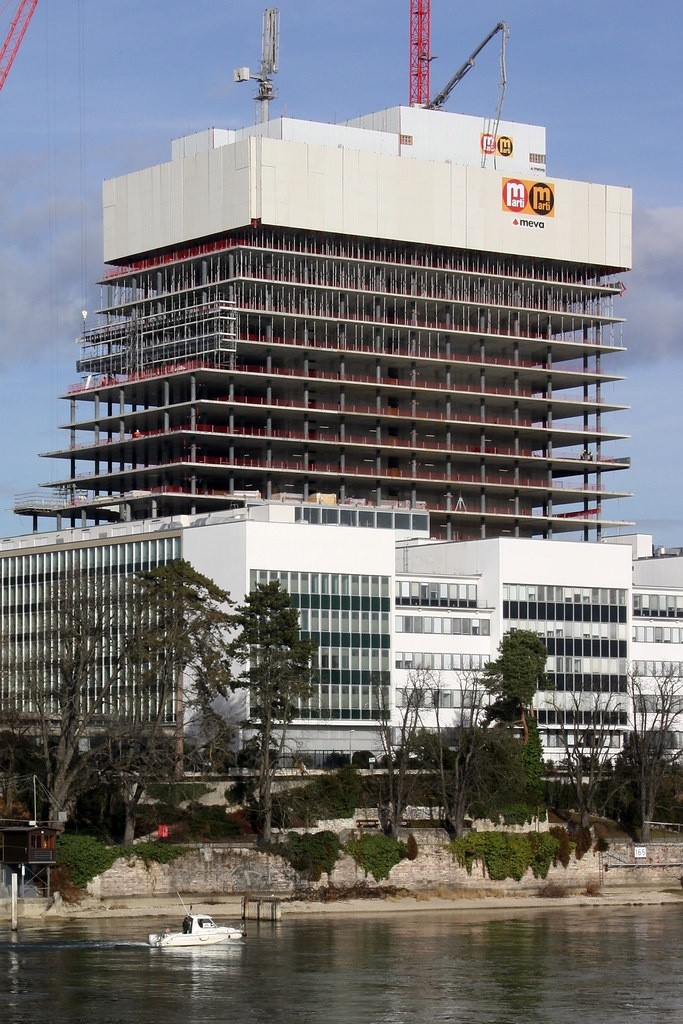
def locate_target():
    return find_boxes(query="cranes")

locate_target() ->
[408,0,432,109]
[0,0,38,92]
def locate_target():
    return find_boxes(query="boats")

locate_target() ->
[148,914,246,947]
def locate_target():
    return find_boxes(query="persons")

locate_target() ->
[182,917,190,934]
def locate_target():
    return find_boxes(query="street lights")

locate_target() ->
[349,730,355,765]
